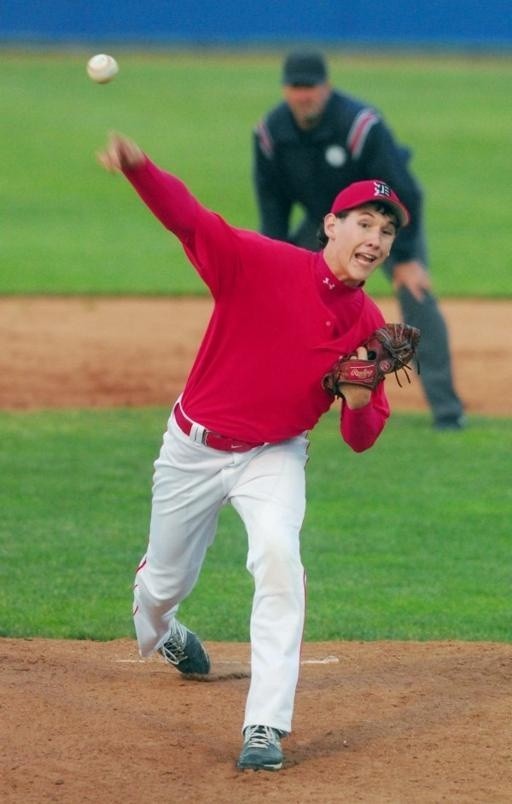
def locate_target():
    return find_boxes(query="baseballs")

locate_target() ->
[87,54,117,84]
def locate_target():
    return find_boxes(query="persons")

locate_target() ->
[92,126,410,771]
[250,49,467,430]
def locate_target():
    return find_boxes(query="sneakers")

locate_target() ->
[236,726,284,770]
[157,616,210,675]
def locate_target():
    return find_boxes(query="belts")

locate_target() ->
[174,404,263,452]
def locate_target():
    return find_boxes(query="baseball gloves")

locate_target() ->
[322,323,420,398]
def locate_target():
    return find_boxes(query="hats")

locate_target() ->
[283,49,325,86]
[330,179,410,228]
